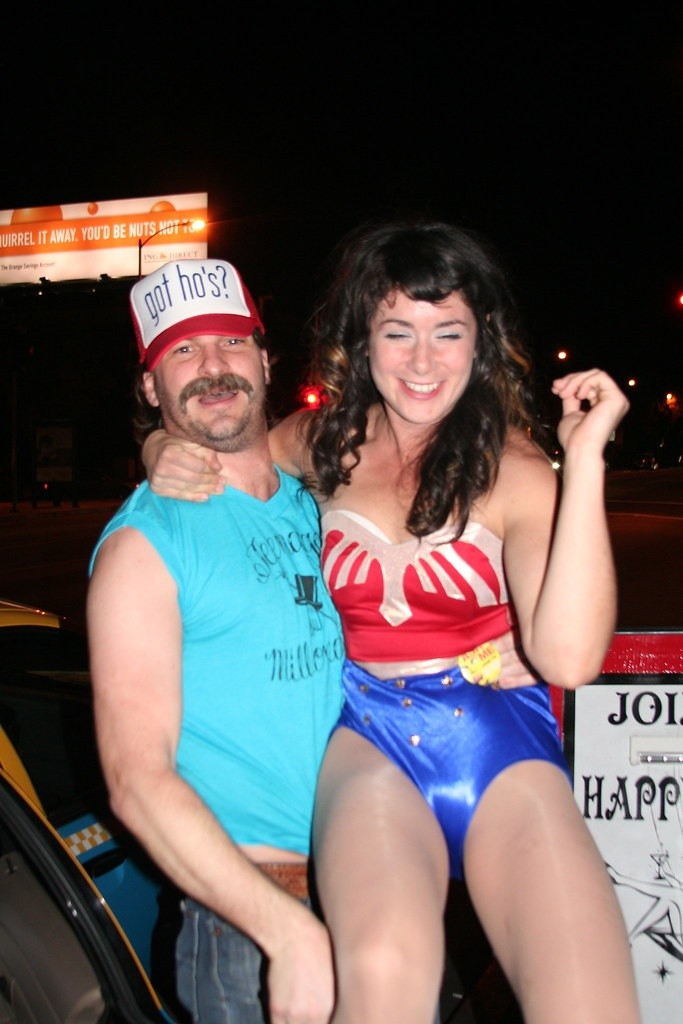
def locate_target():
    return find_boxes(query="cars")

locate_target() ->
[0,598,529,1024]
[631,453,657,470]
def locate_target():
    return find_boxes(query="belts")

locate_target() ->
[257,862,314,898]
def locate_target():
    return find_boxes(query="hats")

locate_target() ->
[128,259,265,372]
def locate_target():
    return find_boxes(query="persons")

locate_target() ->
[86,255,537,1024]
[143,219,645,1024]
